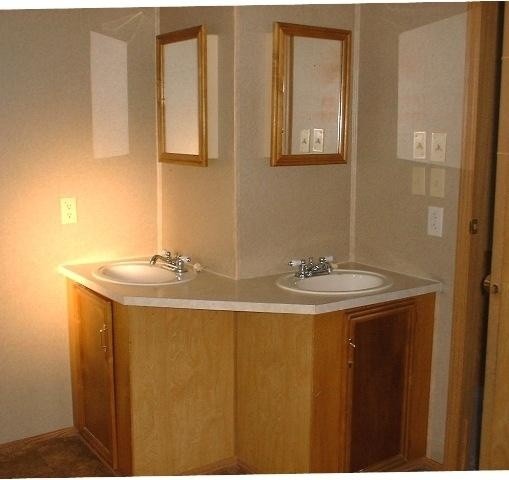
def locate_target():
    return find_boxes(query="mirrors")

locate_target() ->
[156,25,207,167]
[270,22,352,167]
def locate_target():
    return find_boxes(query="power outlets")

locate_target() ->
[411,167,426,196]
[413,132,426,161]
[60,198,78,225]
[430,168,445,199]
[427,206,443,237]
[431,133,447,163]
[313,129,324,153]
[299,129,310,153]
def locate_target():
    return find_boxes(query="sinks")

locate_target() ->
[93,258,198,286]
[282,266,394,296]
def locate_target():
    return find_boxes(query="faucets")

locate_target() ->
[149,250,192,271]
[288,254,334,279]
[174,272,186,281]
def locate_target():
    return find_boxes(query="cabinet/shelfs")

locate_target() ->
[236,292,436,471]
[66,278,235,476]
